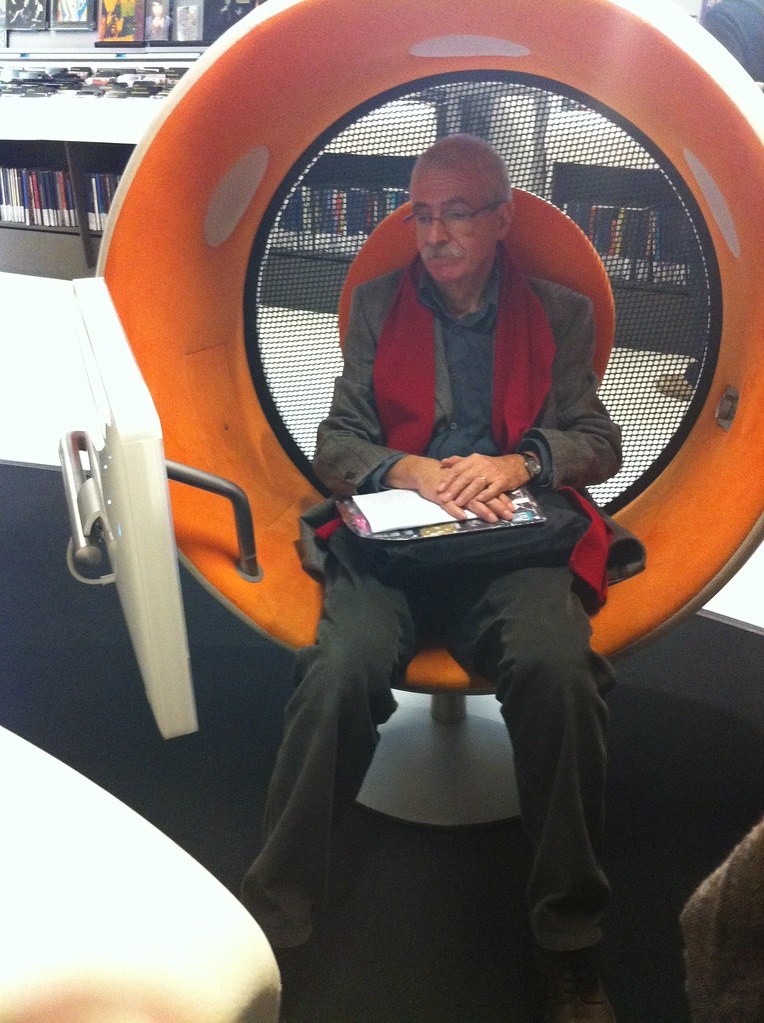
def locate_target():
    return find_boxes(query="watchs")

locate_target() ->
[521,452,541,478]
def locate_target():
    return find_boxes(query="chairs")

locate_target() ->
[96,0,763,824]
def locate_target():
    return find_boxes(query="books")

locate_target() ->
[560,200,693,287]
[82,170,124,231]
[273,183,417,255]
[0,164,79,227]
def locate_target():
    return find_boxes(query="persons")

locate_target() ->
[240,136,645,1023]
[146,0,171,40]
[654,0,764,401]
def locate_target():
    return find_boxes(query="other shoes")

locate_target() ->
[527,934,616,1023]
[271,946,296,983]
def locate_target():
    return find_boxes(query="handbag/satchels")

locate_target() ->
[334,488,547,564]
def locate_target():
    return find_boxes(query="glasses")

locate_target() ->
[402,201,499,233]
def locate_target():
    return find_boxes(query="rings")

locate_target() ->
[480,476,488,486]
